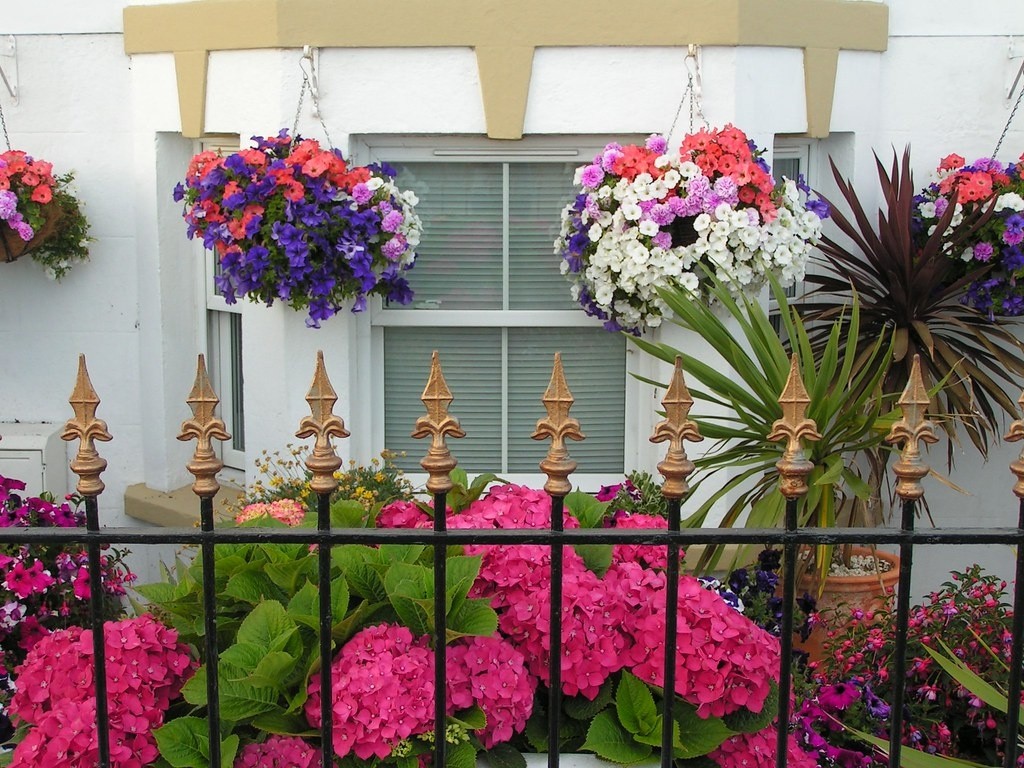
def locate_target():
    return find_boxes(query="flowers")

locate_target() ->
[553,122,831,337]
[0,149,100,285]
[172,127,424,329]
[0,440,1024,768]
[911,151,1024,322]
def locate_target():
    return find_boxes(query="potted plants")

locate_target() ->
[621,250,963,662]
